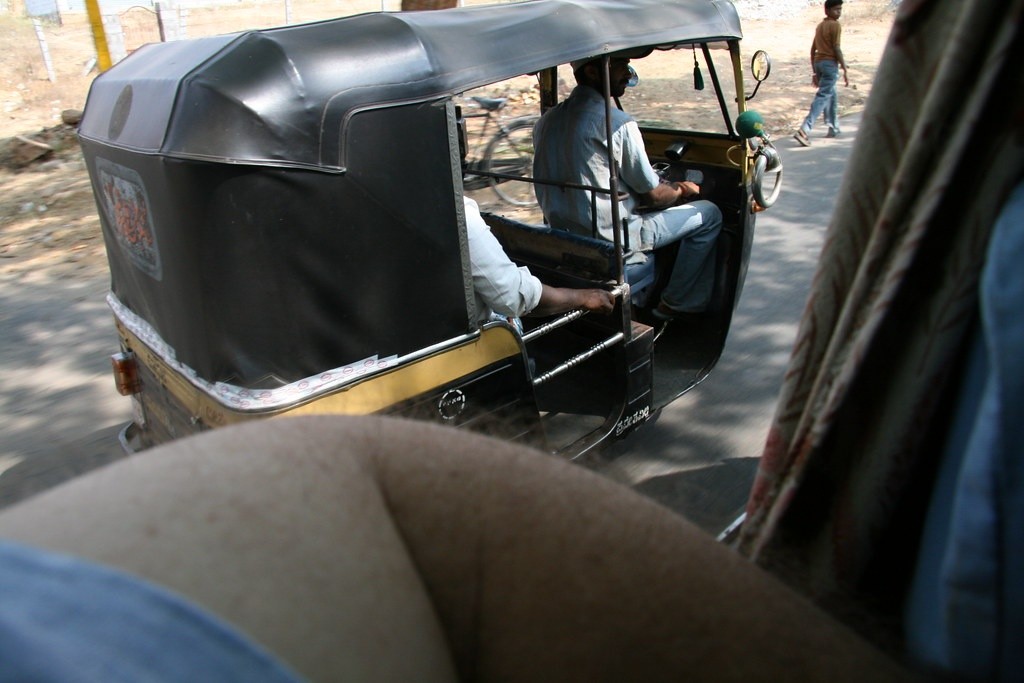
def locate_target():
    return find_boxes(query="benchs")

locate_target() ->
[479,211,655,294]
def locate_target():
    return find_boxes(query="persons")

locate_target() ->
[531,49,723,329]
[0,415,913,683]
[793,0,849,147]
[463,195,616,337]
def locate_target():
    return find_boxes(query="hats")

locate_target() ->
[570,45,654,74]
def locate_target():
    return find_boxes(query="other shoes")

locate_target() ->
[794,128,811,146]
[827,131,843,138]
[652,304,675,318]
[632,290,648,308]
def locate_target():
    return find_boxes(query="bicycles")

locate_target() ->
[462,95,548,207]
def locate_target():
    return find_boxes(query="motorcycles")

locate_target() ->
[76,0,783,467]
